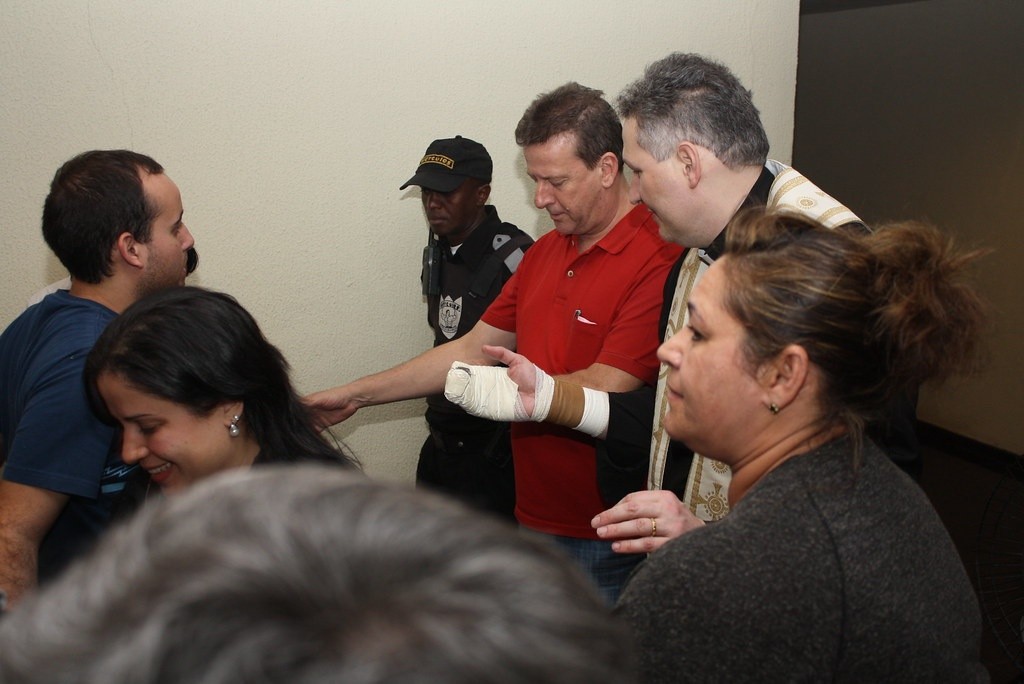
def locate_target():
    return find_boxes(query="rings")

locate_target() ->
[651,518,656,536]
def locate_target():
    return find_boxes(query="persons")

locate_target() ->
[83,287,360,494]
[300,81,684,604]
[443,51,918,558]
[606,207,988,684]
[0,458,635,684]
[399,135,537,531]
[0,148,200,615]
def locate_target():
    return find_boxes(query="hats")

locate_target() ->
[400,134,493,193]
